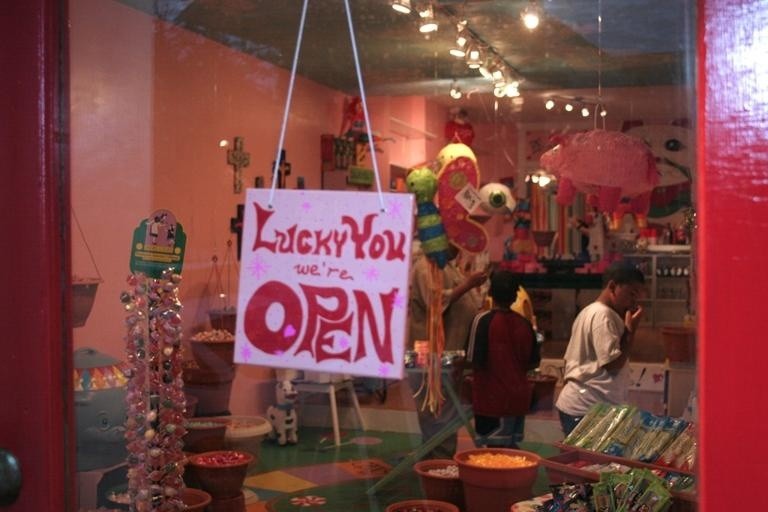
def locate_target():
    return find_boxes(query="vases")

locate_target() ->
[384,448,544,512]
[657,323,696,369]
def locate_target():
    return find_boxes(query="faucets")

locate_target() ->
[665,231,673,243]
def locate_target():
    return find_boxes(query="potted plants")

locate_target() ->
[68,206,105,329]
[153,239,274,512]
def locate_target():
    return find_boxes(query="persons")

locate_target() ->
[408,239,543,462]
[556,256,646,437]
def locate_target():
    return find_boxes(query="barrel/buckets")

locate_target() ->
[659,323,692,361]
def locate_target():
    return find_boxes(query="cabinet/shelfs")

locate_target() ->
[619,255,694,328]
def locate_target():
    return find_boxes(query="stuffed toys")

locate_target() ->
[262,379,299,446]
[339,96,662,274]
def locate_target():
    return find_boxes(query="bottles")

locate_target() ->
[664,222,685,245]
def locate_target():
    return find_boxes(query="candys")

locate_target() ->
[390,453,535,512]
[187,395,259,464]
[108,268,186,512]
[193,328,234,340]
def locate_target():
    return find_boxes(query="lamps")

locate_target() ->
[389,0,439,34]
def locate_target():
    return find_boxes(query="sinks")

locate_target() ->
[646,243,691,252]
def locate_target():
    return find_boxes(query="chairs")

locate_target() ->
[275,368,368,449]
